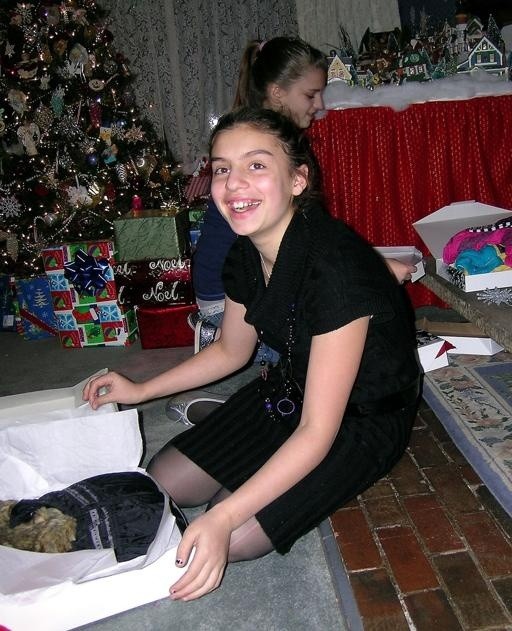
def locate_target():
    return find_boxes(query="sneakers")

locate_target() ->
[165,391,230,427]
[187,312,202,330]
[195,321,217,355]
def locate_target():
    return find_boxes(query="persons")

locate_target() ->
[81,103,423,603]
[187,32,416,365]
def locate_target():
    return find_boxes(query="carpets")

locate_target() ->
[416,333,512,519]
[0,332,365,631]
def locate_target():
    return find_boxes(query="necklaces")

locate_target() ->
[259,253,273,279]
[256,301,296,425]
